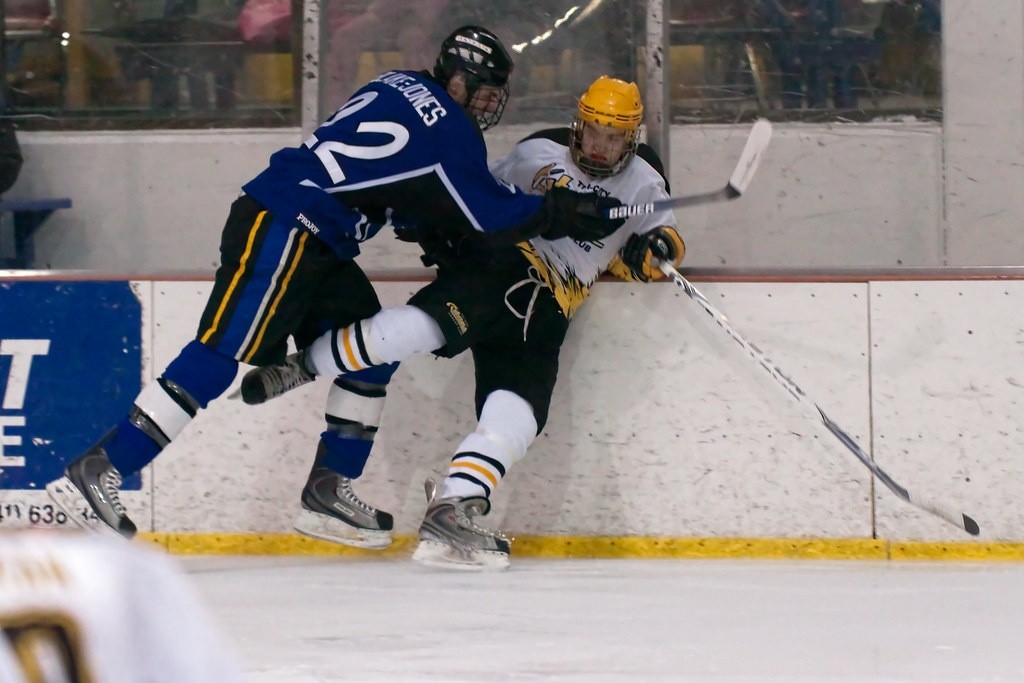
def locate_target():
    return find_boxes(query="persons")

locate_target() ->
[41,28,630,543]
[313,0,459,113]
[0,524,246,683]
[229,74,683,571]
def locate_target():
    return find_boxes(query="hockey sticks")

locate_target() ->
[609,118,775,220]
[654,256,980,538]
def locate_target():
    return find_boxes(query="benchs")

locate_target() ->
[0,198,72,269]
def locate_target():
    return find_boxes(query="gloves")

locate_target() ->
[538,187,628,242]
[631,223,686,282]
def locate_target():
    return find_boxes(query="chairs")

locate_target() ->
[0,0,865,128]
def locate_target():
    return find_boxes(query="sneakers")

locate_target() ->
[46,449,137,540]
[411,479,510,571]
[293,465,393,550]
[228,347,317,406]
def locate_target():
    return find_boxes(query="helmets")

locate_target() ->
[567,75,645,144]
[432,25,514,98]
